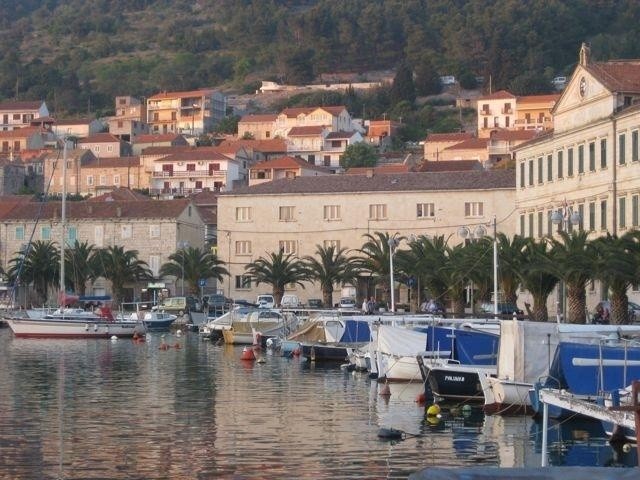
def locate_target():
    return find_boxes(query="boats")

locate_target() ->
[41,306,103,323]
[202,306,640,422]
[119,309,178,330]
[0,279,19,328]
[27,306,61,321]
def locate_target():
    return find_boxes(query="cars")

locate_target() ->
[479,292,525,321]
[416,300,446,320]
[191,294,232,314]
[304,299,325,312]
[590,301,640,325]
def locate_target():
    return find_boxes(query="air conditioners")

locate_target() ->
[197,161,204,165]
[177,161,183,166]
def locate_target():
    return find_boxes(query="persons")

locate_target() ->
[362,296,377,315]
[84,301,115,322]
[592,306,610,324]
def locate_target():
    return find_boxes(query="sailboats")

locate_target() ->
[5,136,148,341]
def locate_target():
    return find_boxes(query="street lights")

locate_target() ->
[551,197,580,323]
[387,235,401,316]
[457,224,486,321]
[407,233,432,315]
[191,102,199,133]
[175,240,191,296]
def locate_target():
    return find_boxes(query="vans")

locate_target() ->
[440,76,455,85]
[256,294,277,311]
[151,296,195,318]
[280,294,299,313]
[551,76,567,85]
[335,296,358,313]
